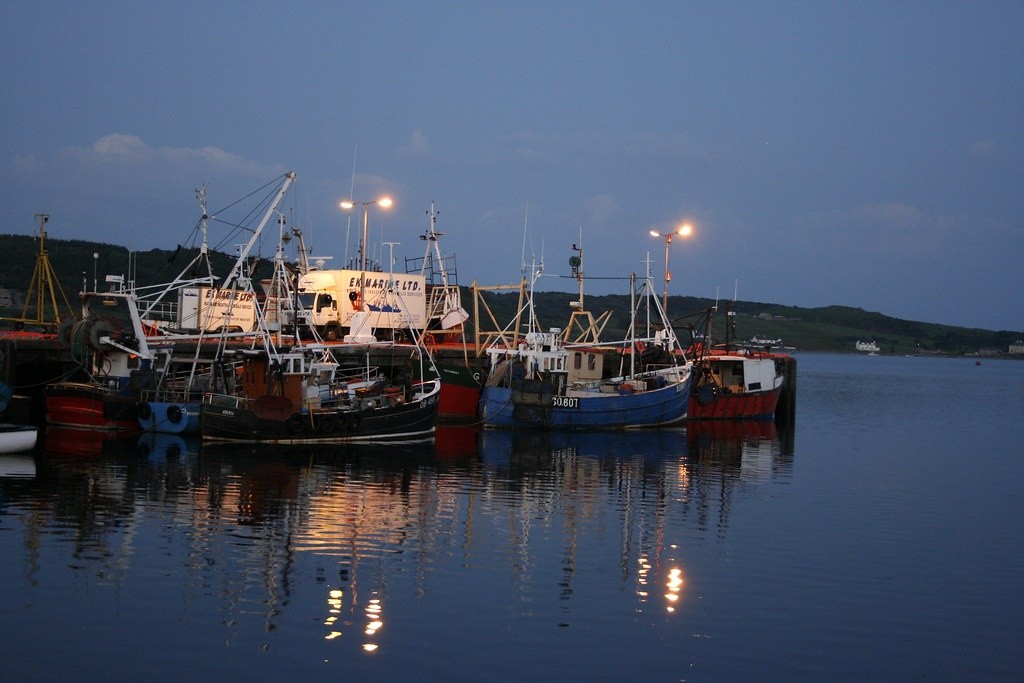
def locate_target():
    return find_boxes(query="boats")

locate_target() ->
[42,149,495,454]
[473,206,699,434]
[681,274,798,420]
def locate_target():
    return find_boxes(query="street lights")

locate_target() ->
[650,228,691,317]
[343,198,392,311]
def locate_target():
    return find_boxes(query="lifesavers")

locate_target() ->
[306,413,320,433]
[349,412,363,431]
[288,412,306,432]
[166,405,182,423]
[334,412,349,430]
[320,414,335,433]
[137,401,151,420]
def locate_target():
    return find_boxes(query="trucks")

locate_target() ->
[294,269,426,343]
